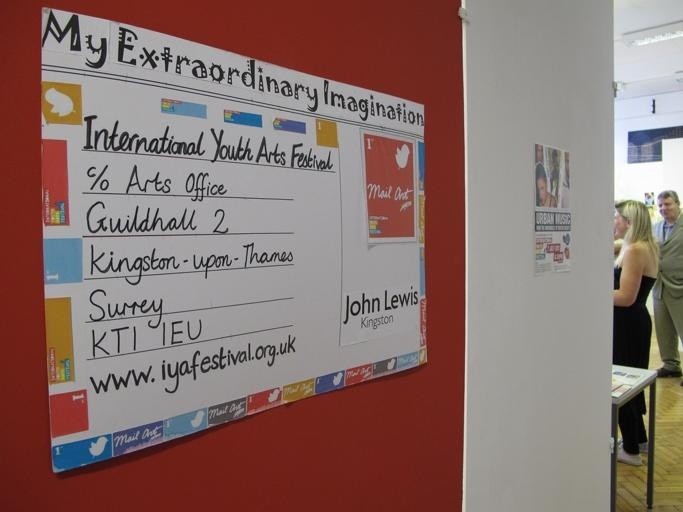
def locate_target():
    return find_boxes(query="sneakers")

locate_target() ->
[617,438,648,465]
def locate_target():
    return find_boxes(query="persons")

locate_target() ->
[651,190,683,387]
[613,199,658,466]
[536,163,557,208]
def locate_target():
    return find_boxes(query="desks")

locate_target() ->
[608,362,659,511]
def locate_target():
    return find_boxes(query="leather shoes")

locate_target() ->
[655,366,682,379]
